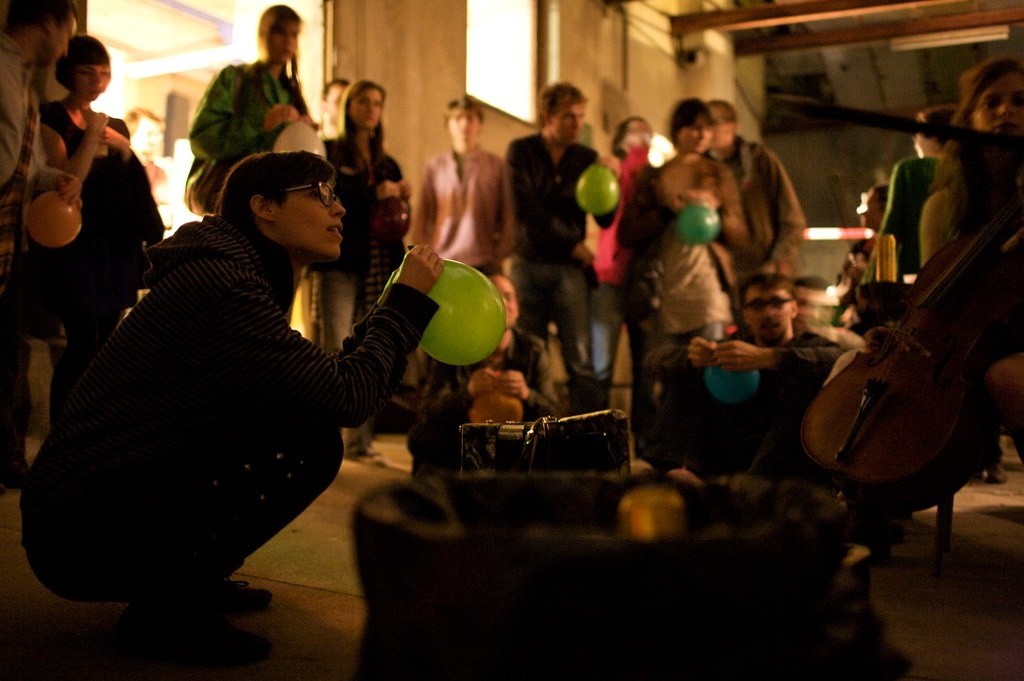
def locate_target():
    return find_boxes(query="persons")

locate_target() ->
[429,261,561,421]
[647,271,844,479]
[619,98,746,347]
[704,101,807,327]
[593,116,653,284]
[0,0,76,493]
[188,5,320,327]
[323,80,413,465]
[506,82,625,416]
[20,136,441,665]
[831,54,1024,484]
[413,94,515,414]
[41,34,166,436]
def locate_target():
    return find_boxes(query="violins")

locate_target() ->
[800,186,1024,506]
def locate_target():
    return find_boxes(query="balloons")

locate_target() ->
[704,366,759,403]
[576,163,619,216]
[470,370,523,424]
[371,196,411,238]
[419,258,506,365]
[27,181,82,247]
[678,203,721,243]
[272,116,325,158]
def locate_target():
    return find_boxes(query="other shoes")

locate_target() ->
[983,464,1008,481]
[112,608,273,668]
[215,577,272,612]
[347,441,380,457]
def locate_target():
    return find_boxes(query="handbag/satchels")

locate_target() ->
[370,197,411,242]
[184,66,229,217]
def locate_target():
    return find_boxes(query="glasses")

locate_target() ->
[280,179,343,208]
[743,294,794,311]
